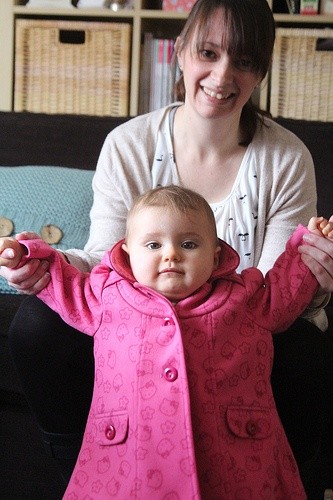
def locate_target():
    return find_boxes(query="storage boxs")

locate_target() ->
[268,26,333,125]
[11,16,130,118]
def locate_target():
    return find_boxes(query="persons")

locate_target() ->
[0,183,332,499]
[0,0,332,485]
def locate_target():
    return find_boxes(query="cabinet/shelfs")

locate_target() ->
[0,0,333,125]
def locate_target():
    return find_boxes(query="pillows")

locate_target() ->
[1,163,96,291]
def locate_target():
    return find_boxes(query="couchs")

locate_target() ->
[0,108,333,500]
